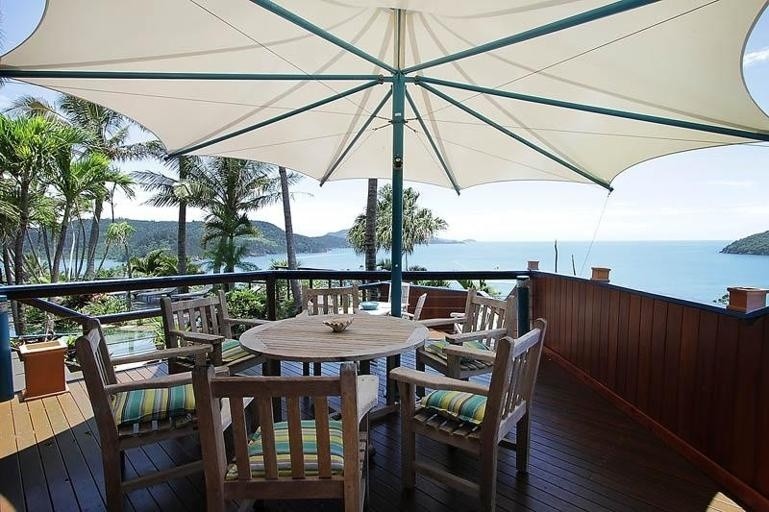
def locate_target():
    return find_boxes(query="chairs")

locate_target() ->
[188,365,381,512]
[78,318,256,512]
[390,318,548,512]
[411,287,521,401]
[300,285,372,404]
[397,292,427,323]
[159,290,279,440]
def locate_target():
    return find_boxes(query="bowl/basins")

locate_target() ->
[360,302,379,310]
[323,318,355,333]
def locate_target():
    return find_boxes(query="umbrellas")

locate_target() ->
[0,0,769,405]
